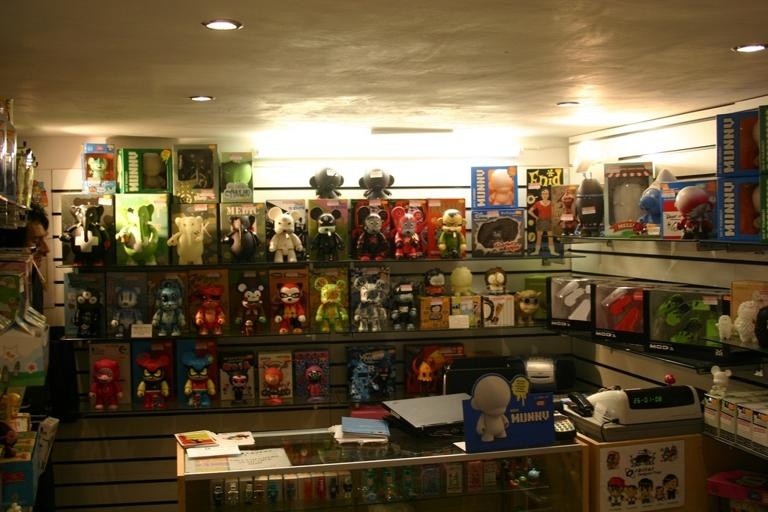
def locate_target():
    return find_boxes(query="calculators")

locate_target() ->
[550,414,576,440]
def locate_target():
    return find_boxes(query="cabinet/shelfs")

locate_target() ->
[175,417,590,512]
[542,234,768,374]
[56,255,587,419]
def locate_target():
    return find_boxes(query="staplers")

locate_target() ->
[568,391,593,417]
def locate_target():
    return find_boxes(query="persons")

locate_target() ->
[4,202,51,316]
